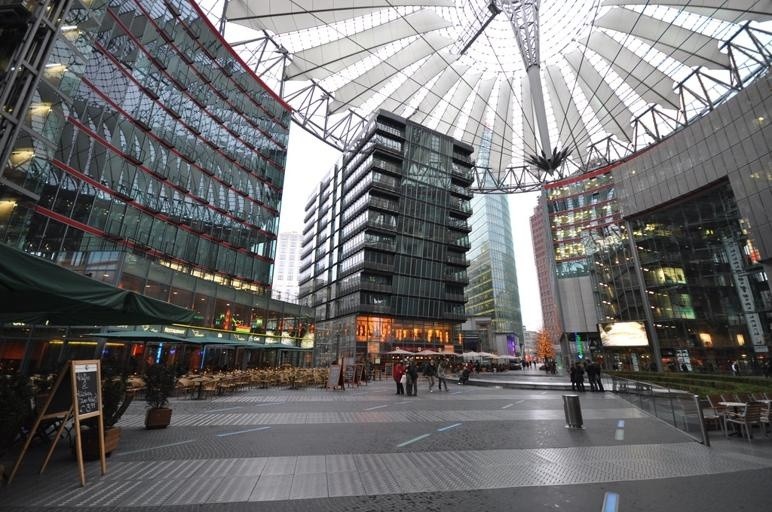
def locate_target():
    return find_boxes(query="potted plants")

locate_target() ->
[73,362,134,459]
[140,364,177,429]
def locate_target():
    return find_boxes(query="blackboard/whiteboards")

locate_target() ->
[357,365,363,382]
[385,363,393,378]
[345,365,357,384]
[70,359,103,420]
[327,365,341,386]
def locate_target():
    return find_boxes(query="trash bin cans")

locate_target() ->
[563,395,584,430]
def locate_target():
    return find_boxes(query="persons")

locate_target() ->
[570,357,605,392]
[458,359,481,385]
[545,359,556,374]
[522,360,531,367]
[392,355,449,396]
[727,359,741,376]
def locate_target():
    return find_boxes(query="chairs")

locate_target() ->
[706,390,772,444]
[111,364,344,398]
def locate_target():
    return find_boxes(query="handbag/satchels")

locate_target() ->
[400,375,406,383]
